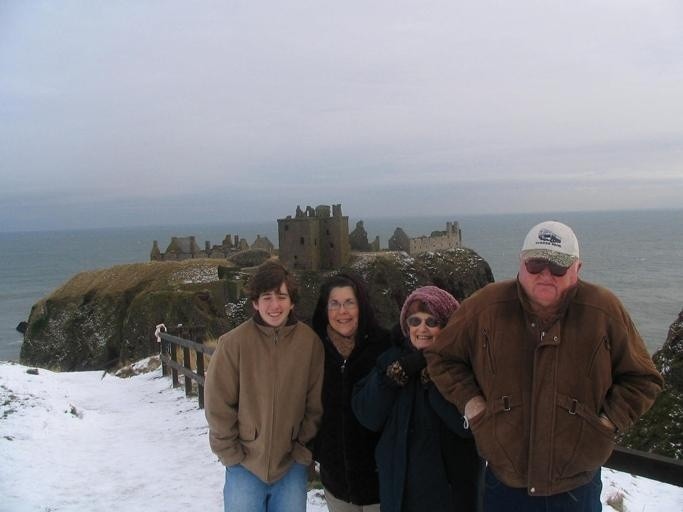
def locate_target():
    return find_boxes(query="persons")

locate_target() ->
[420,220,665,512]
[347,285,487,511]
[303,270,395,512]
[201,263,329,512]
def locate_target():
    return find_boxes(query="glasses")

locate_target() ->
[406,317,441,328]
[525,259,569,279]
[326,300,360,310]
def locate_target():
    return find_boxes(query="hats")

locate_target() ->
[399,286,460,338]
[522,220,580,268]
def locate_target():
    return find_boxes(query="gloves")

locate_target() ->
[398,348,428,374]
